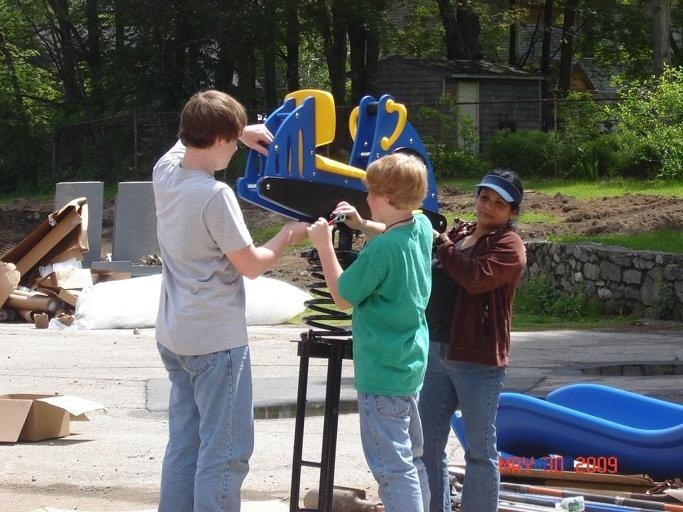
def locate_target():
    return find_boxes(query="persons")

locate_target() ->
[417,166,530,510]
[305,149,437,512]
[148,89,315,512]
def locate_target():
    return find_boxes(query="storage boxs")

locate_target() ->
[0,394,105,444]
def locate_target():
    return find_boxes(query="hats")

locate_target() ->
[473,173,521,203]
